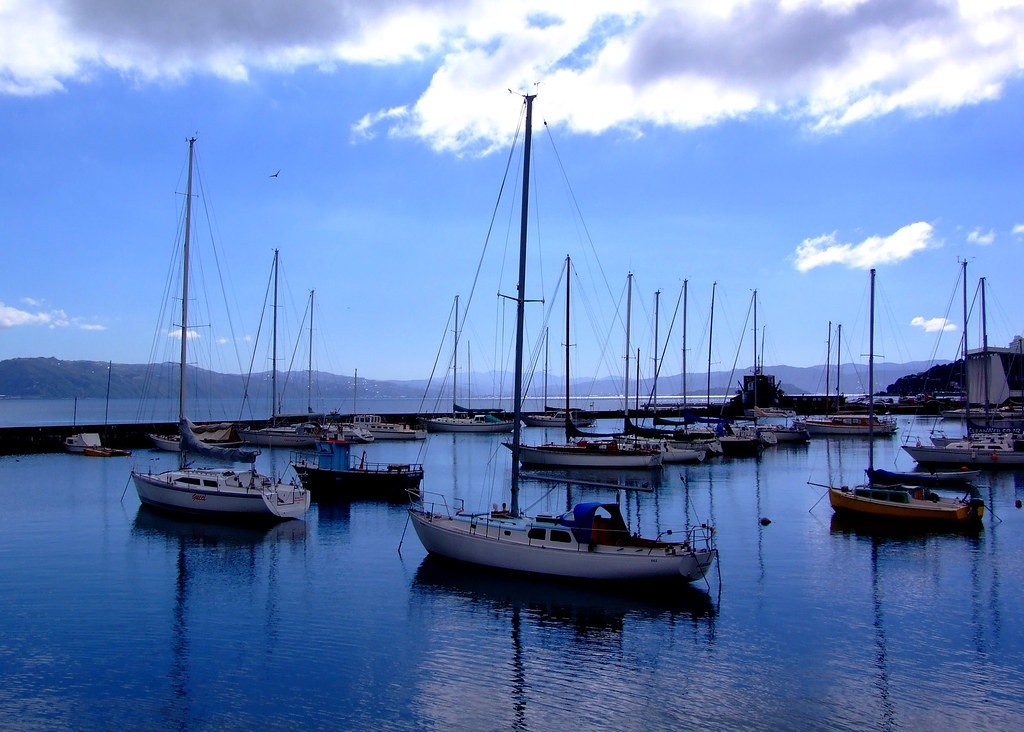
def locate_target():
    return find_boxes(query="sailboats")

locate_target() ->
[132,135,312,519]
[827,268,985,526]
[151,247,1023,502]
[404,81,718,585]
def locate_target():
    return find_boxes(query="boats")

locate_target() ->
[83,447,131,458]
[64,432,101,454]
